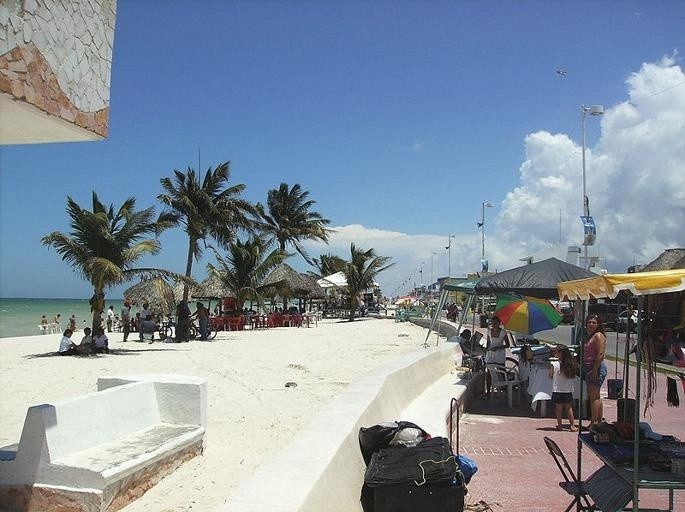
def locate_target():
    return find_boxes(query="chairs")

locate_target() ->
[543,435,598,512]
[109,309,324,332]
[487,363,522,409]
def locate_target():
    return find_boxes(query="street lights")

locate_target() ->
[477,200,496,315]
[394,251,437,300]
[580,104,604,269]
[445,234,456,283]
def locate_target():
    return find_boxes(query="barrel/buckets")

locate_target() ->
[608,371,623,400]
[616,388,635,423]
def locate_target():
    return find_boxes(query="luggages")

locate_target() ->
[361,437,465,512]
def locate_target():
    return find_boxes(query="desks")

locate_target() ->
[578,429,685,512]
[525,354,590,420]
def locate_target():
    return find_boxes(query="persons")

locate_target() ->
[484,315,510,399]
[518,344,532,378]
[577,313,608,430]
[549,345,579,431]
[459,328,486,357]
[37,297,211,356]
[408,299,417,312]
[400,299,409,311]
[211,305,308,328]
[446,302,461,323]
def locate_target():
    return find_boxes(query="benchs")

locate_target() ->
[41,376,208,511]
[0,375,142,511]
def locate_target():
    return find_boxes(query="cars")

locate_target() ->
[487,305,497,317]
[478,303,489,314]
[616,310,656,334]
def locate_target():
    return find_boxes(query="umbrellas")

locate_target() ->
[394,296,425,315]
[190,270,241,318]
[473,258,603,300]
[253,262,349,311]
[121,274,178,327]
[492,291,564,337]
[167,274,209,312]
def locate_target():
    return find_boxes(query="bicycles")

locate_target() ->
[159,315,218,341]
